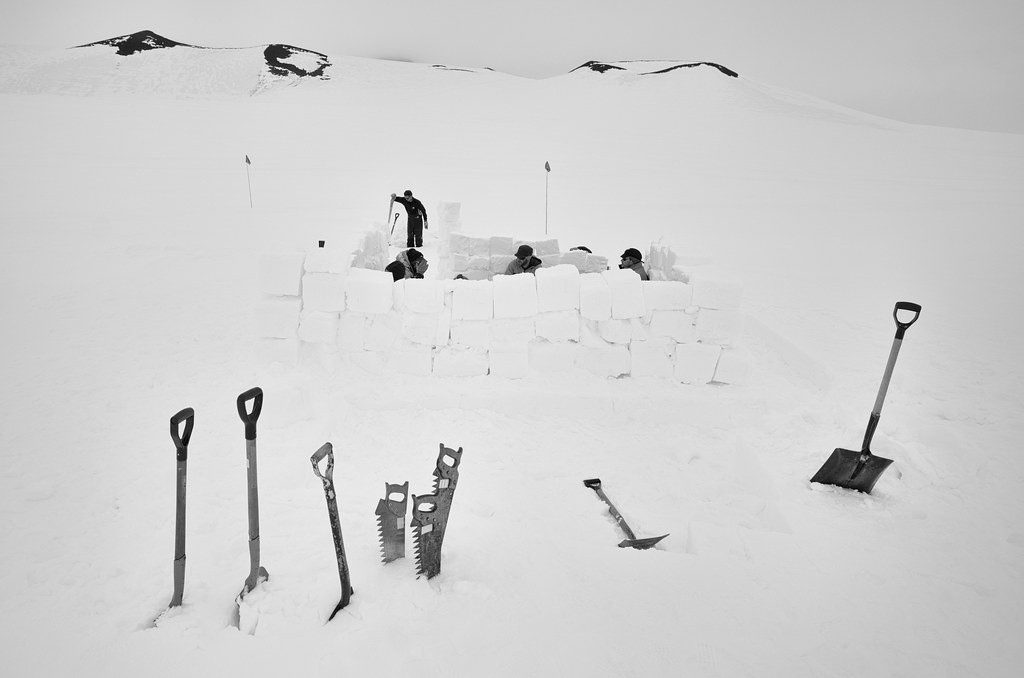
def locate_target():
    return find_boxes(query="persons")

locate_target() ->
[618,248,649,280]
[391,190,429,248]
[384,248,429,283]
[504,245,542,275]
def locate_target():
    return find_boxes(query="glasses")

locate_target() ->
[405,198,410,200]
[622,258,633,261]
[518,257,527,260]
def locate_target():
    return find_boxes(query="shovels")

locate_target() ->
[811,301,921,496]
[582,476,671,550]
[152,406,197,628]
[235,387,269,630]
[311,442,354,623]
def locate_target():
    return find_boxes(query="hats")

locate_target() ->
[620,248,642,261]
[385,261,405,282]
[407,248,421,260]
[514,245,533,259]
